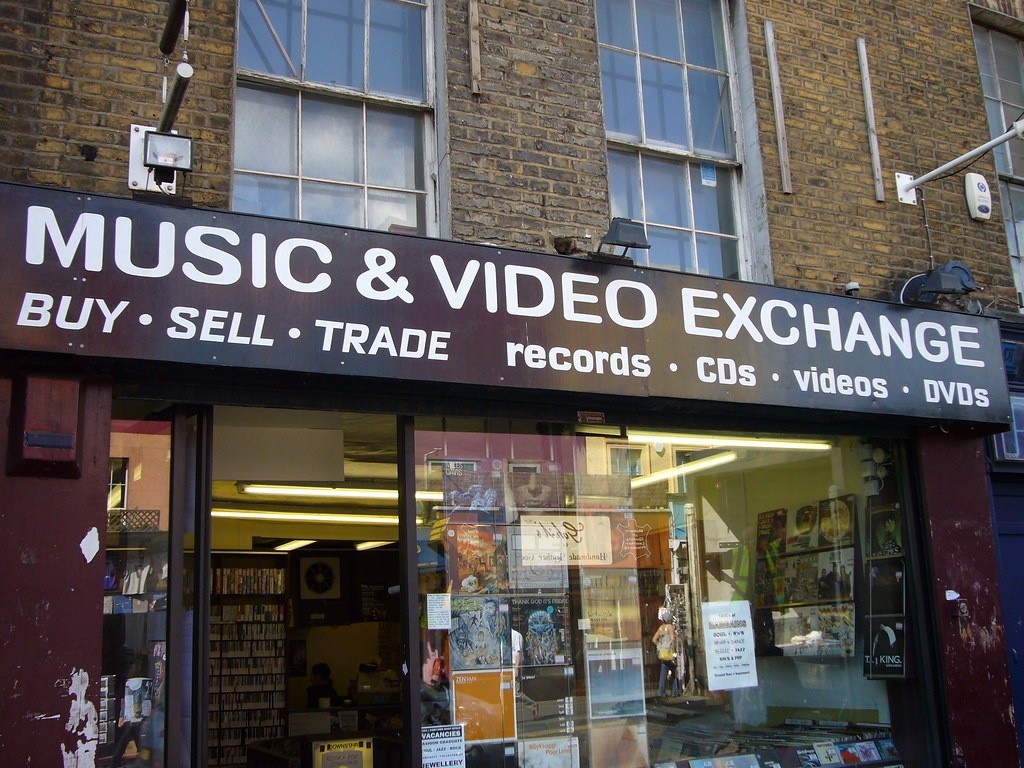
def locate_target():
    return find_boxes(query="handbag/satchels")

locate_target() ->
[659,624,677,660]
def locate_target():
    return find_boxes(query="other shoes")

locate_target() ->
[657,694,668,698]
[673,694,681,697]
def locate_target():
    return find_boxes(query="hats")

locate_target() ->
[662,613,673,623]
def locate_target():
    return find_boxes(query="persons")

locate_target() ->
[816,549,855,602]
[498,613,539,721]
[287,663,338,713]
[457,599,506,665]
[652,608,682,699]
[108,651,149,768]
[506,472,558,523]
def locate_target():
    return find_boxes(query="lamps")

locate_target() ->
[914,273,965,310]
[142,130,193,182]
[586,218,652,266]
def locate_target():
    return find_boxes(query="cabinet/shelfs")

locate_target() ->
[208,554,291,768]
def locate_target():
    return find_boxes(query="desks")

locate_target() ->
[247,723,413,768]
